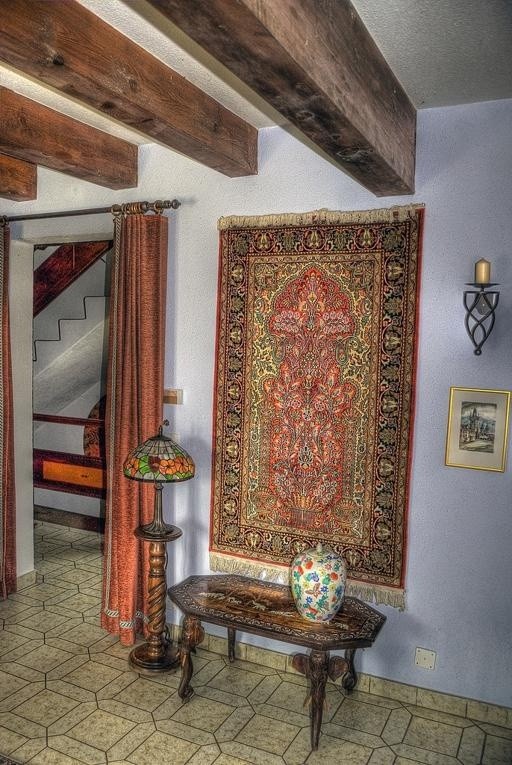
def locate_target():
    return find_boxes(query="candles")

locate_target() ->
[475,257,492,283]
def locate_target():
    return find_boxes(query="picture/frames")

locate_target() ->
[443,387,512,474]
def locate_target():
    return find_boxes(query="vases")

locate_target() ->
[290,539,348,624]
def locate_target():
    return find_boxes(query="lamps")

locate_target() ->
[120,421,196,677]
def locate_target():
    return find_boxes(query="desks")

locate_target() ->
[161,572,386,751]
